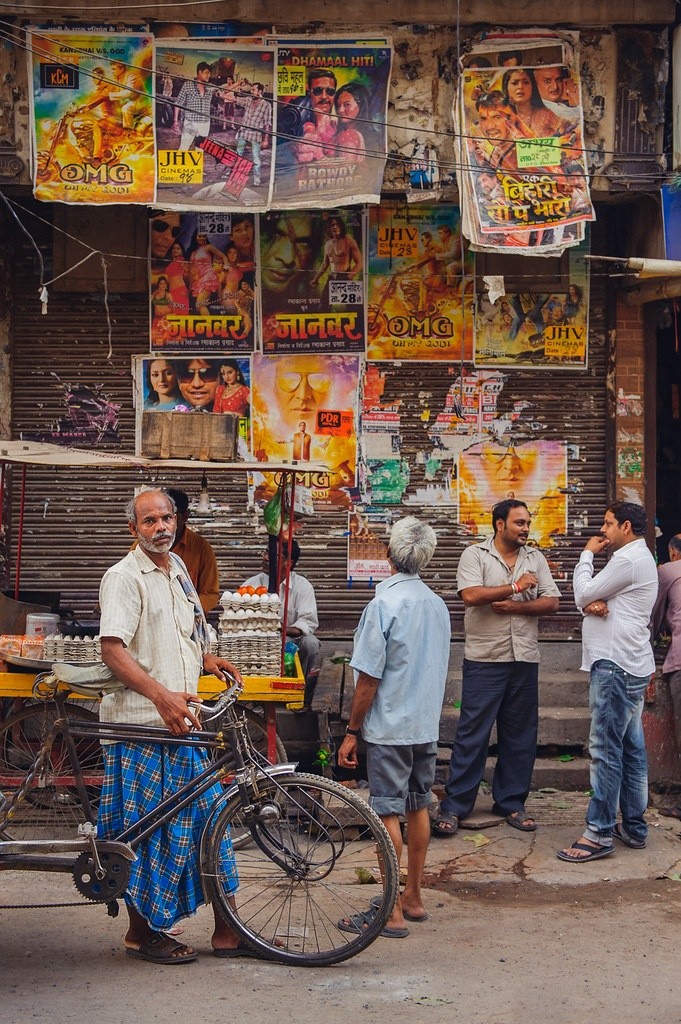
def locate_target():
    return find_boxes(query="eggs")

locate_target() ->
[237,585,267,595]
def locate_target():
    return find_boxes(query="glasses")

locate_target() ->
[151,218,183,238]
[176,366,219,383]
[260,550,286,560]
[312,85,337,96]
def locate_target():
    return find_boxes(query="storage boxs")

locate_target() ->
[140,410,237,463]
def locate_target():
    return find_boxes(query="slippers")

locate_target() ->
[214,931,292,960]
[126,932,199,964]
[339,905,411,938]
[556,841,617,862]
[610,822,648,848]
[371,895,428,923]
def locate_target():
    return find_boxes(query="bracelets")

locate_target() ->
[509,582,520,593]
[345,725,361,735]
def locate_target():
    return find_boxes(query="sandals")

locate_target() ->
[433,809,458,834]
[506,808,537,831]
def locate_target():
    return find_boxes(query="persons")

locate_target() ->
[26,25,589,490]
[240,537,322,714]
[336,515,452,938]
[557,502,659,863]
[650,534,681,817]
[426,499,562,839]
[126,488,220,619]
[94,491,287,965]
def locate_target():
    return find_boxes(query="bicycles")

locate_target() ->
[0,694,291,851]
[0,663,399,969]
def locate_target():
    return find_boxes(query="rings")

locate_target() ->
[595,606,598,609]
